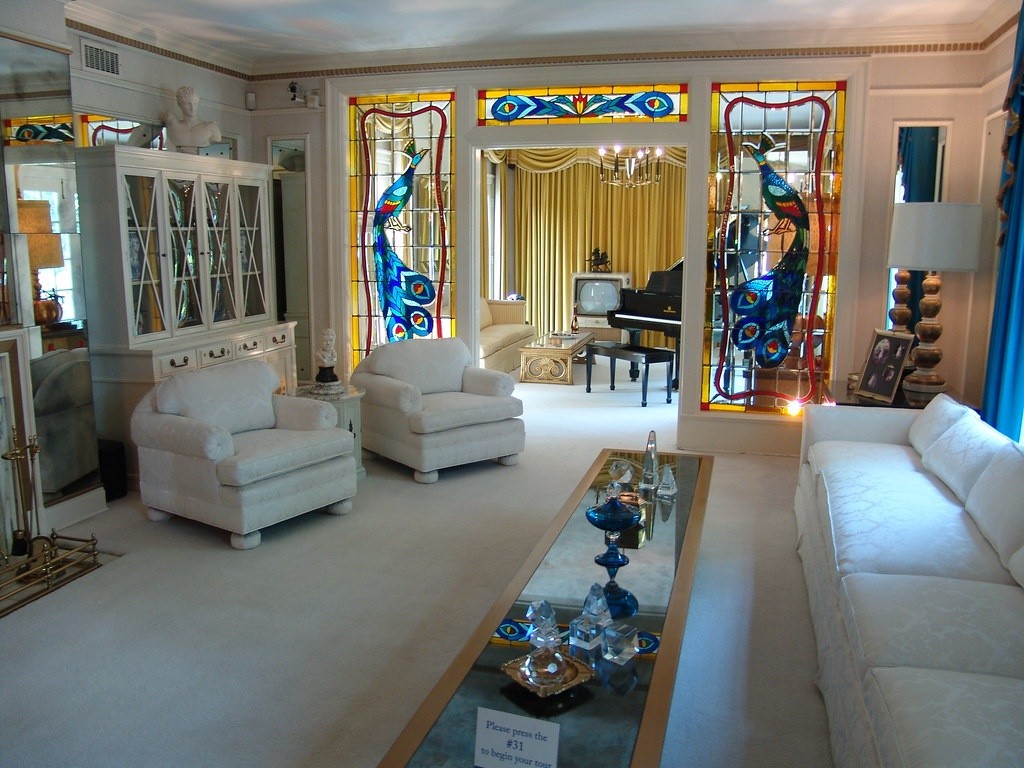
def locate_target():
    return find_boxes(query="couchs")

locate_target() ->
[427,297,536,374]
[130,358,360,550]
[349,336,526,484]
[30,348,99,501]
[793,404,1024,768]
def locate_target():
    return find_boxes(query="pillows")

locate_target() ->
[920,409,1013,505]
[964,440,1024,569]
[907,392,981,456]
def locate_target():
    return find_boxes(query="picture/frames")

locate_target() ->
[853,327,915,404]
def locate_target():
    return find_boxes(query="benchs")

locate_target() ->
[584,341,675,407]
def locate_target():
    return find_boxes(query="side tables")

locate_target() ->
[294,385,367,481]
[821,379,982,416]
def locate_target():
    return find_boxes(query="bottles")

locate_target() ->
[571,303,579,334]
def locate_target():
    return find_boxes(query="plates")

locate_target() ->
[551,335,575,339]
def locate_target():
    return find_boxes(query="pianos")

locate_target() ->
[605,214,767,392]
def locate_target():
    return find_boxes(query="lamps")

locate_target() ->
[27,233,64,302]
[886,202,984,393]
[17,200,53,234]
[598,144,664,191]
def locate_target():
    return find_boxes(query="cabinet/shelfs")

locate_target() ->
[278,172,311,380]
[73,145,297,491]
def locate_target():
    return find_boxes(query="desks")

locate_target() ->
[577,317,630,344]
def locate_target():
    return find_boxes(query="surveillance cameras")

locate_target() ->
[289,80,301,94]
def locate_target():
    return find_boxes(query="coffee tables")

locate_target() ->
[375,448,715,768]
[517,331,597,386]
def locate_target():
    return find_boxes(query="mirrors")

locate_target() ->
[266,132,315,385]
[885,120,955,370]
[0,37,104,509]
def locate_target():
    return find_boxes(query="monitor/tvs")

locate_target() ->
[572,272,632,328]
